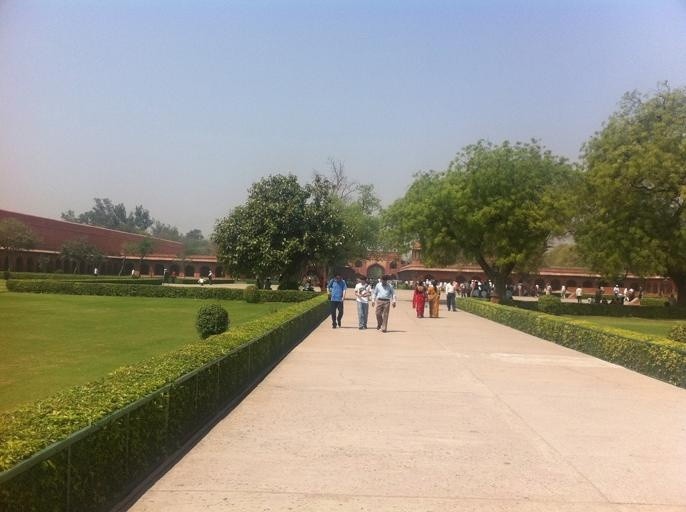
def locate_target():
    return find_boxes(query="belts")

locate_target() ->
[377,299,389,300]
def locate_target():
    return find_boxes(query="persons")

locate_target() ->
[131,269,135,275]
[445,279,457,311]
[254,278,265,290]
[163,268,169,283]
[93,267,98,276]
[412,280,428,318]
[327,273,347,328]
[365,277,645,305]
[170,271,177,284]
[264,278,272,290]
[668,294,676,305]
[371,276,397,333]
[354,275,372,330]
[302,279,315,292]
[197,277,206,285]
[208,270,213,285]
[427,280,441,318]
